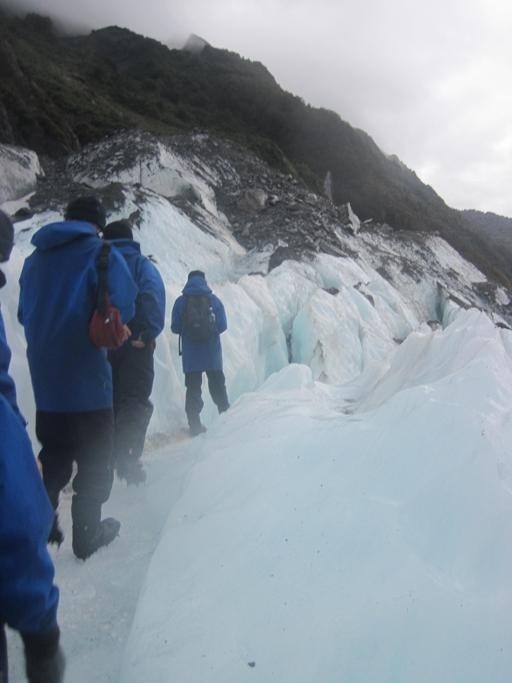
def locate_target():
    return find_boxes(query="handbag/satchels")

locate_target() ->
[89,306,128,348]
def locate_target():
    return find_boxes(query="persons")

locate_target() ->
[15,194,139,557]
[0,209,69,682]
[99,218,165,487]
[171,269,232,435]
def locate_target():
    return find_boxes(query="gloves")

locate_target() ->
[21,628,64,683]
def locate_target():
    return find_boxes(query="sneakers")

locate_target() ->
[188,426,207,436]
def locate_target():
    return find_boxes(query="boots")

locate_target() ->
[118,461,147,485]
[72,511,121,559]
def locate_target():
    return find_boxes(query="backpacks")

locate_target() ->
[182,295,216,341]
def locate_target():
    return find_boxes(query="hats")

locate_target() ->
[0,210,14,262]
[64,197,106,231]
[102,222,133,240]
